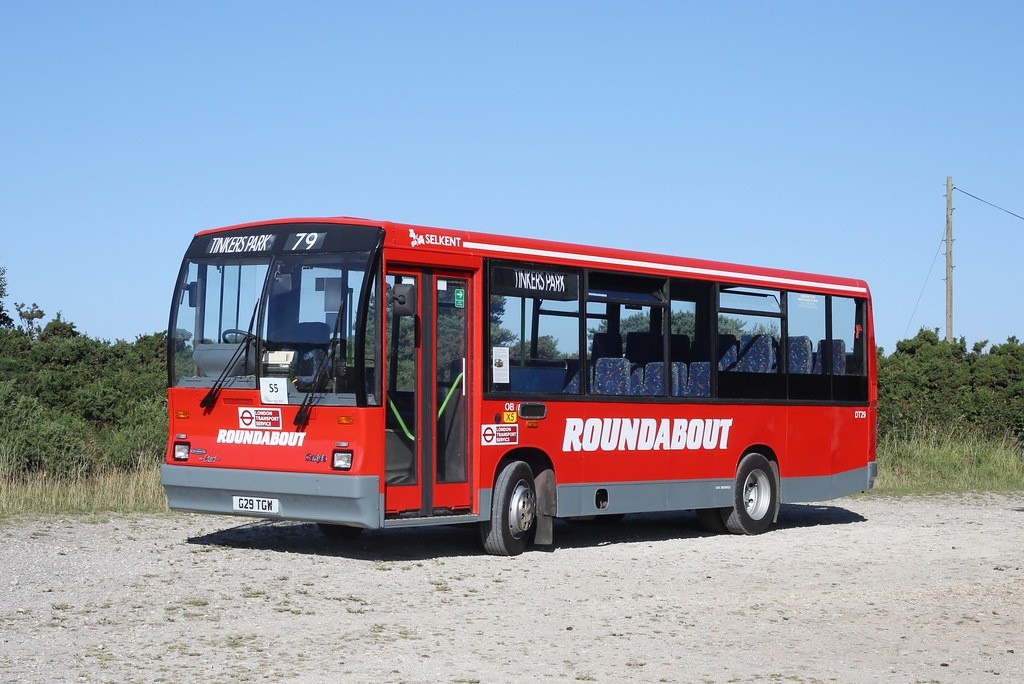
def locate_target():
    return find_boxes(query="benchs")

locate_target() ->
[490,355,567,392]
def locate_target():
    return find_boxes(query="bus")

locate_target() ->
[162,216,878,556]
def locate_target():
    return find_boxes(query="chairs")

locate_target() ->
[563,358,594,394]
[739,335,772,373]
[779,336,814,374]
[641,362,688,396]
[631,363,644,395]
[626,332,659,362]
[813,339,846,375]
[593,357,630,395]
[659,333,690,362]
[687,362,723,396]
[719,334,738,371]
[290,322,330,377]
[591,332,622,365]
